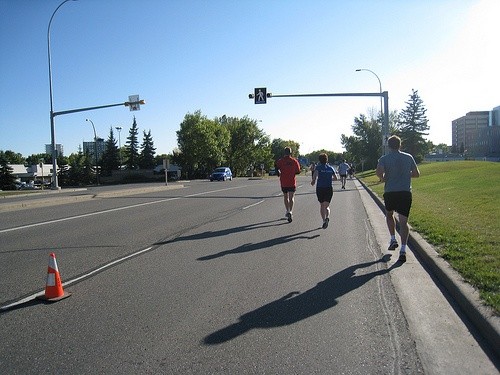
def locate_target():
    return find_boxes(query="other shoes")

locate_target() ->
[287,212,292,223]
[323,218,329,229]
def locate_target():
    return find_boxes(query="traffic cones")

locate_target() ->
[35,253,73,303]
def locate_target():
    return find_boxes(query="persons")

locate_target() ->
[275,147,301,222]
[304,159,355,190]
[376,136,420,262]
[311,153,338,229]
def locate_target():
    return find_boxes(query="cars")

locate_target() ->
[210,167,233,182]
[269,168,277,176]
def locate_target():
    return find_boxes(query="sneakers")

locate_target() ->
[388,239,399,250]
[399,251,406,262]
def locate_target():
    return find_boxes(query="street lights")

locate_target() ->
[85,118,100,185]
[356,68,383,129]
